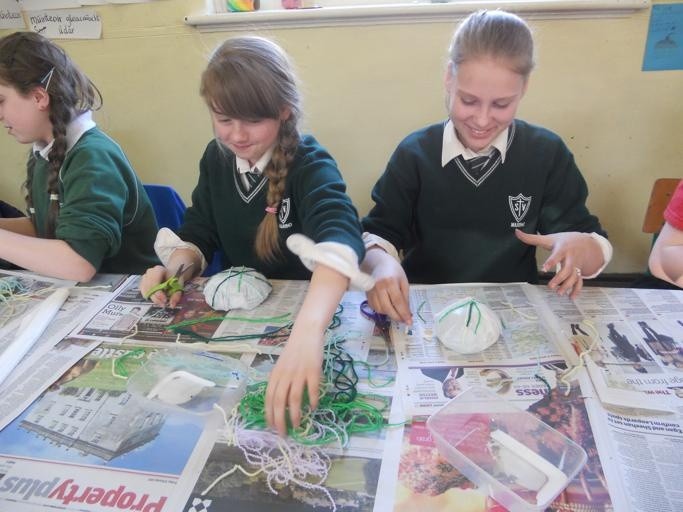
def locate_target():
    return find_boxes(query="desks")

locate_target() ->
[2,279,681,511]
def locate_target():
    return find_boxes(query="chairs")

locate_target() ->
[642,179,681,289]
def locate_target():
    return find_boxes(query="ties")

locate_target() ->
[246,172,262,190]
[468,156,489,177]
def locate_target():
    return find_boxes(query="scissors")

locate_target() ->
[144,261,196,303]
[360,300,392,348]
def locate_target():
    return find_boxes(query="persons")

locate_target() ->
[421,368,464,399]
[573,321,683,373]
[0,31,164,282]
[139,35,366,440]
[658,180,683,282]
[359,11,613,326]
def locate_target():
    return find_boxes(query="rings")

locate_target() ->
[576,267,581,276]
